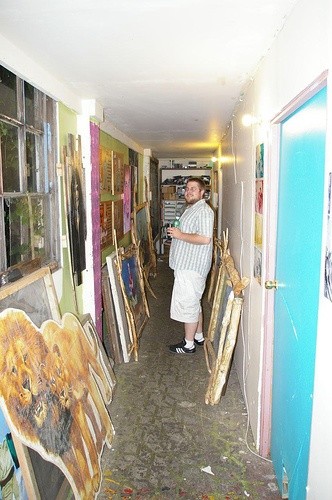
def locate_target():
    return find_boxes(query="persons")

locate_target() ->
[168,178,215,356]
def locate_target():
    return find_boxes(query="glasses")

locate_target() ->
[185,187,201,191]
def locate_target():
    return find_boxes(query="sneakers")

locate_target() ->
[194,338,205,347]
[169,338,196,354]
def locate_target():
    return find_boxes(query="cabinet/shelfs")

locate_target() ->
[158,167,213,255]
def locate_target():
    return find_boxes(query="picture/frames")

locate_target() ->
[105,246,135,363]
[100,266,124,365]
[114,248,150,345]
[133,201,156,276]
[66,165,86,273]
[0,265,75,500]
[77,313,118,408]
[203,227,248,406]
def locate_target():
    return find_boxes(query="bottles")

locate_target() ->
[173,217,180,238]
[166,220,173,240]
